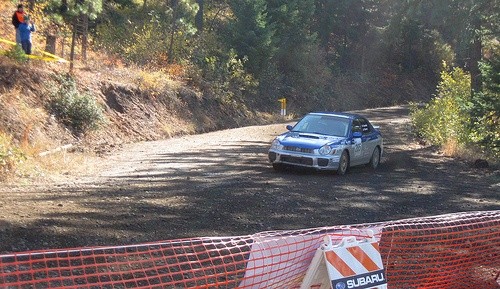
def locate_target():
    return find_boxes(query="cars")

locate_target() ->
[268,111,384,175]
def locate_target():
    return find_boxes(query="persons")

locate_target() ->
[19,15,35,54]
[12,3,27,46]
